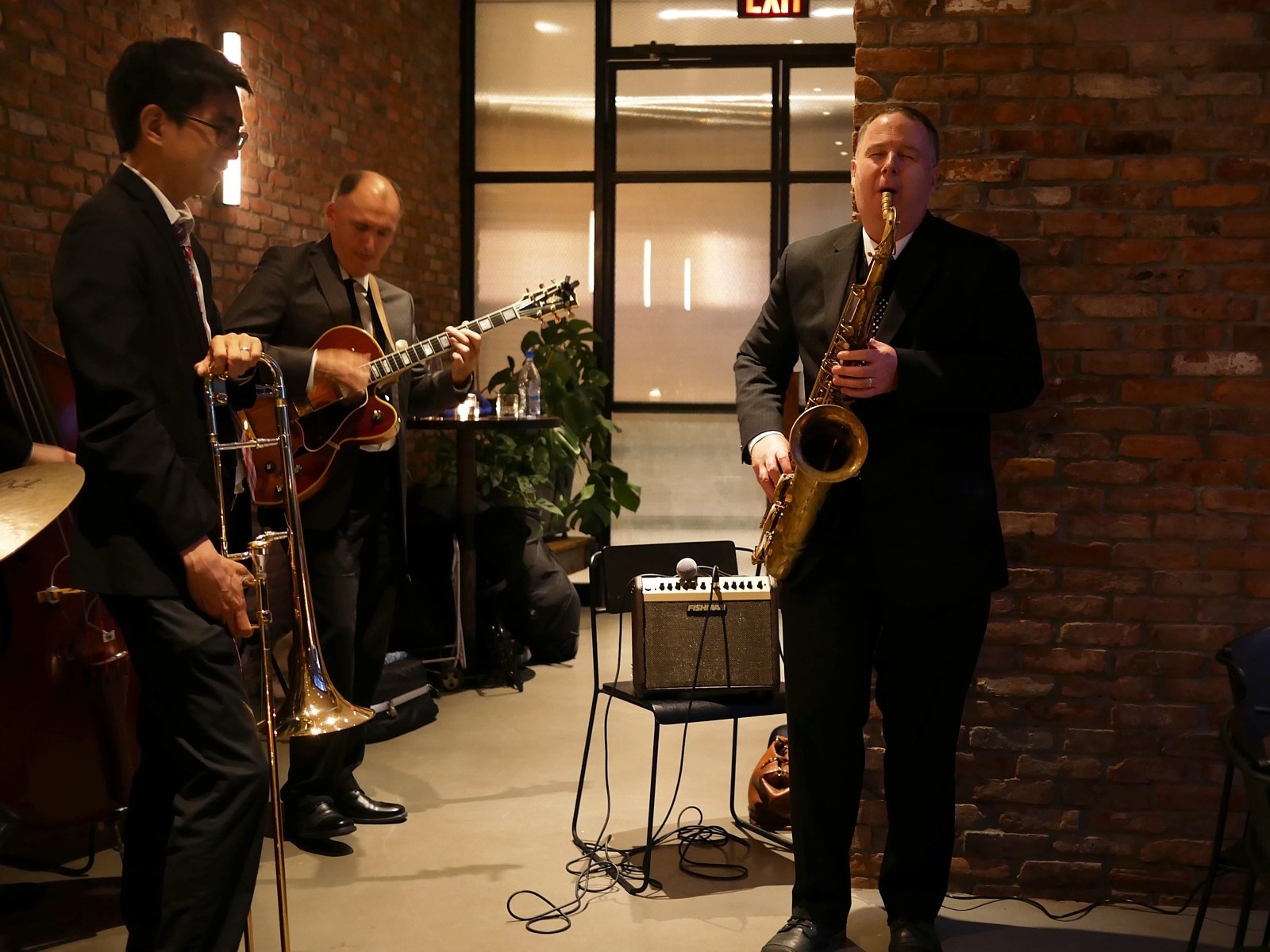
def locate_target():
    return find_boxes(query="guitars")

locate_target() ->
[238,274,582,507]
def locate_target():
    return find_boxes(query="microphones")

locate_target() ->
[675,556,697,579]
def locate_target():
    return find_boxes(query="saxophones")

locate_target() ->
[751,191,900,580]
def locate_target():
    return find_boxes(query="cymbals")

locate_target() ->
[0,461,88,561]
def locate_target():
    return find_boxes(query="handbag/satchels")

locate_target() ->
[354,674,438,742]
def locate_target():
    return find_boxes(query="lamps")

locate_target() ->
[223,32,244,207]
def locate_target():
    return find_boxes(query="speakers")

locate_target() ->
[632,575,780,697]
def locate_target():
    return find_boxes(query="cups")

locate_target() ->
[497,394,520,420]
[461,393,479,421]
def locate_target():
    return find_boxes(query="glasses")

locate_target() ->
[162,107,248,151]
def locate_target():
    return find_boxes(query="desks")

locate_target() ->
[544,534,598,578]
[406,415,561,692]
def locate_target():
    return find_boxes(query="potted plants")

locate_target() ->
[411,315,642,546]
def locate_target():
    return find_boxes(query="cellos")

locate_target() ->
[0,270,141,831]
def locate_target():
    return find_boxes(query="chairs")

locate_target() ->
[1181,624,1270,952]
[572,539,794,894]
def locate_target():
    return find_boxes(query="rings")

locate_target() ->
[240,347,249,350]
[868,378,872,387]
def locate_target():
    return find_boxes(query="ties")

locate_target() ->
[174,222,209,348]
[859,252,896,366]
[342,278,363,329]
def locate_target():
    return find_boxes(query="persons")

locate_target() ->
[0,378,97,941]
[53,39,269,952]
[225,169,482,831]
[733,105,1043,952]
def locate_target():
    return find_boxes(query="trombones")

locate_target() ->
[204,351,372,952]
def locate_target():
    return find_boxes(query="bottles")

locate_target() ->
[518,350,541,420]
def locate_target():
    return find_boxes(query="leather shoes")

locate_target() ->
[286,796,357,839]
[887,922,941,952]
[329,792,408,825]
[760,914,846,952]
